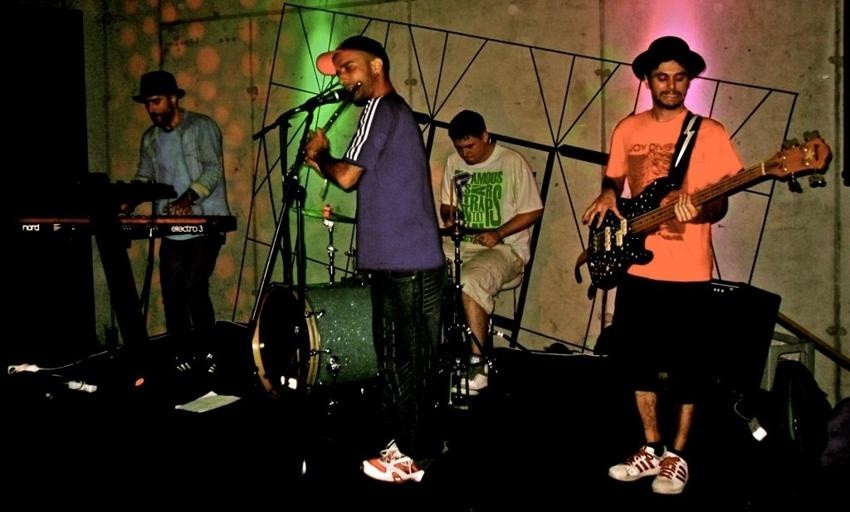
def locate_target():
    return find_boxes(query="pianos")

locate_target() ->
[18,216,238,241]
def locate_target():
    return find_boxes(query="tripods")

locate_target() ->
[409,260,502,417]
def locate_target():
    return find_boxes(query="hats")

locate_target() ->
[315,35,390,77]
[630,35,705,83]
[130,70,186,104]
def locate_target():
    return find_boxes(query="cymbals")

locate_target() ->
[440,225,495,237]
[293,207,356,224]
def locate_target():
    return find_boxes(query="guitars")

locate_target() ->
[586,131,832,290]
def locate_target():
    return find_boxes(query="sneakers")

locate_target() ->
[174,351,197,380]
[651,451,689,495]
[607,445,668,481]
[206,350,219,377]
[358,440,453,484]
[454,352,489,390]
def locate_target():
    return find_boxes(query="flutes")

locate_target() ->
[285,82,362,174]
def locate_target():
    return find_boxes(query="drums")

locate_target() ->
[251,281,379,403]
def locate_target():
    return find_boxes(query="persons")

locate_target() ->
[119,72,231,376]
[580,36,746,496]
[439,109,544,396]
[305,35,448,483]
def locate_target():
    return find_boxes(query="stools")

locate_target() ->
[487,272,534,375]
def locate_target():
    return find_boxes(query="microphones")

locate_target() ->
[303,88,351,106]
[453,171,472,188]
